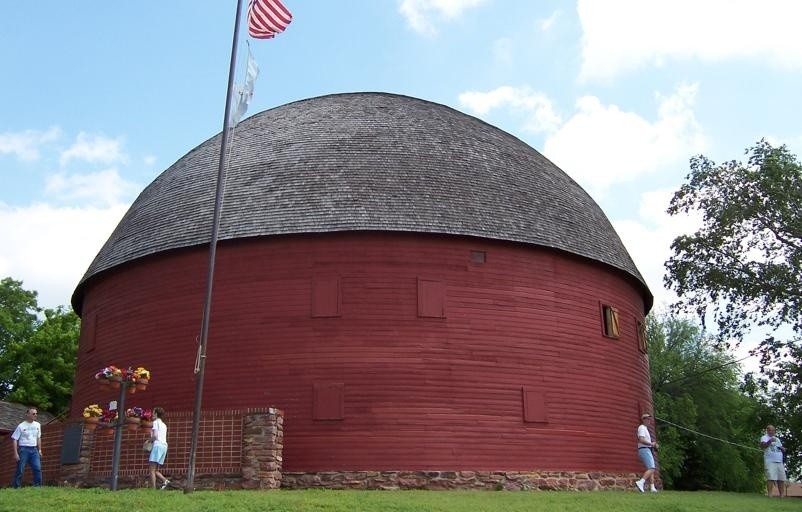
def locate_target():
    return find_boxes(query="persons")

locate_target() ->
[759,425,785,498]
[634,413,658,492]
[11,406,42,488]
[146,406,171,490]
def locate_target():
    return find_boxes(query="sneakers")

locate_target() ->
[161,480,170,489]
[651,488,663,494]
[635,481,645,493]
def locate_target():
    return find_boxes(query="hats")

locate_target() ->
[641,414,652,419]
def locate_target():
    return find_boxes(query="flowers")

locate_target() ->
[89,365,152,387]
[83,400,152,421]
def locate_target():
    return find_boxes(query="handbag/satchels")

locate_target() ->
[142,440,153,453]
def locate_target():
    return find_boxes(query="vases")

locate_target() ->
[99,381,148,395]
[86,416,152,436]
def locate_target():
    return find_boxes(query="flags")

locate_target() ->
[247,0,294,40]
